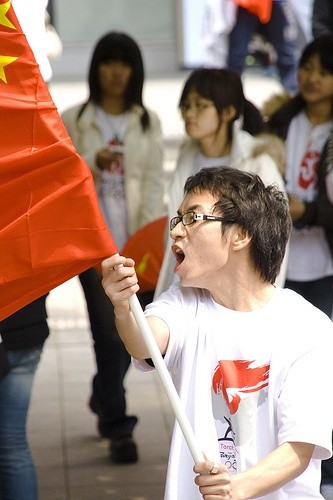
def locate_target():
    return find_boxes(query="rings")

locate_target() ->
[210,463,219,474]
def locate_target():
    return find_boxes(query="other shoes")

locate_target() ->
[110,415,138,464]
[90,384,125,439]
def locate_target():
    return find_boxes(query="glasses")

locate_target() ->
[170,211,236,230]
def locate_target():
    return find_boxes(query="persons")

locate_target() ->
[152,67,293,303]
[100,165,333,500]
[227,0,308,96]
[265,32,333,500]
[0,293,50,500]
[60,30,165,467]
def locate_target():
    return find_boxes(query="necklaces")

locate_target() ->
[103,109,127,145]
[305,111,330,141]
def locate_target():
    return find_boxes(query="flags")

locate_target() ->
[0,0,117,324]
